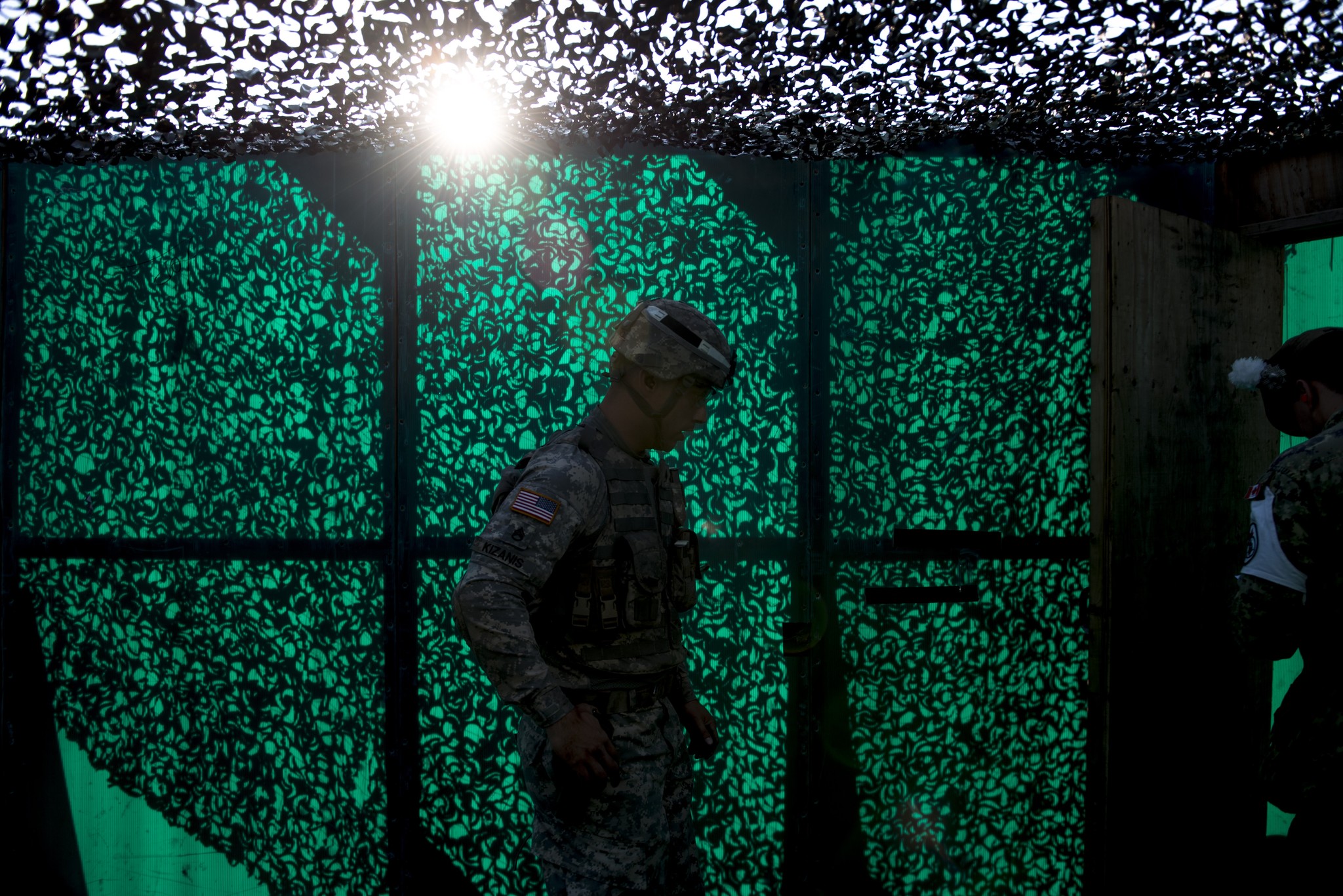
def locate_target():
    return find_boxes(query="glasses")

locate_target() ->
[668,379,717,411]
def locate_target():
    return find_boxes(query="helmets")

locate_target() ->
[608,297,732,390]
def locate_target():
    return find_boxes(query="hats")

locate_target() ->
[1231,325,1343,438]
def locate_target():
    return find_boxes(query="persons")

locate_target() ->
[456,301,739,896]
[1229,327,1342,887]
